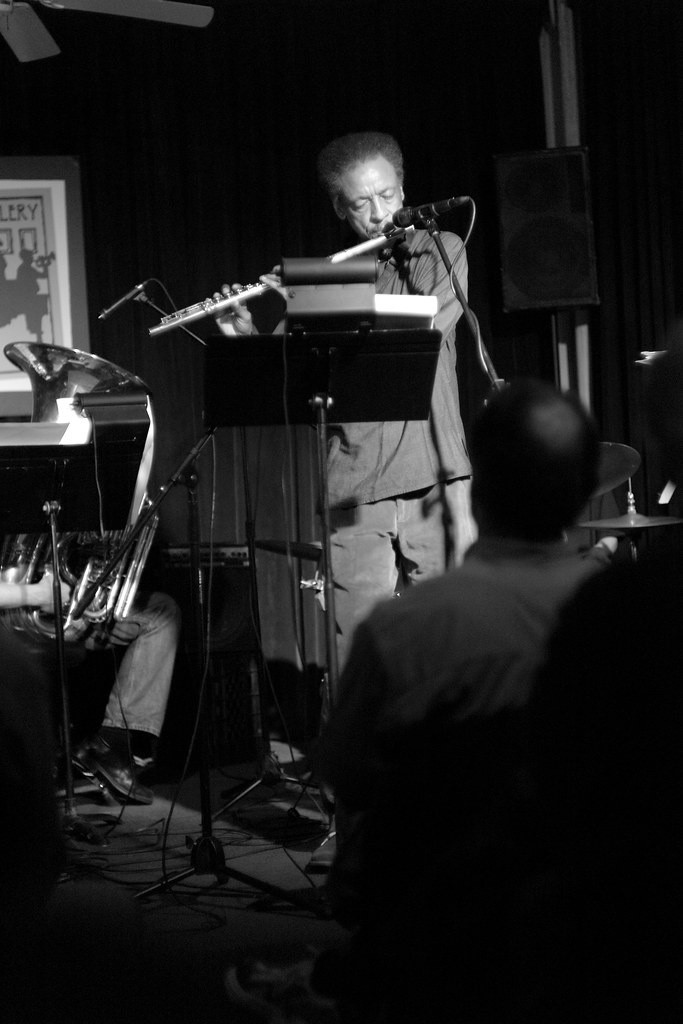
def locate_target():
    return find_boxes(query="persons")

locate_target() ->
[0,572,184,805]
[208,130,481,870]
[1,618,63,1023]
[326,325,683,1024]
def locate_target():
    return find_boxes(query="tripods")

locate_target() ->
[0,426,333,921]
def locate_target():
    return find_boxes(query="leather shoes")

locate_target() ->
[73,726,155,804]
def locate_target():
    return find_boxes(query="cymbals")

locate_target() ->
[575,505,683,533]
[585,438,643,501]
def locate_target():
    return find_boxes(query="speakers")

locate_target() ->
[491,145,602,313]
[142,543,266,778]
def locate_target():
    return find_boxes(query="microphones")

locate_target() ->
[392,195,470,229]
[97,281,149,320]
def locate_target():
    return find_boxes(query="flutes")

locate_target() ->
[144,223,418,339]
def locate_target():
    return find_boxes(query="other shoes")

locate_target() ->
[281,757,324,795]
[310,813,338,865]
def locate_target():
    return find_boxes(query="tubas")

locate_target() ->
[1,338,160,671]
